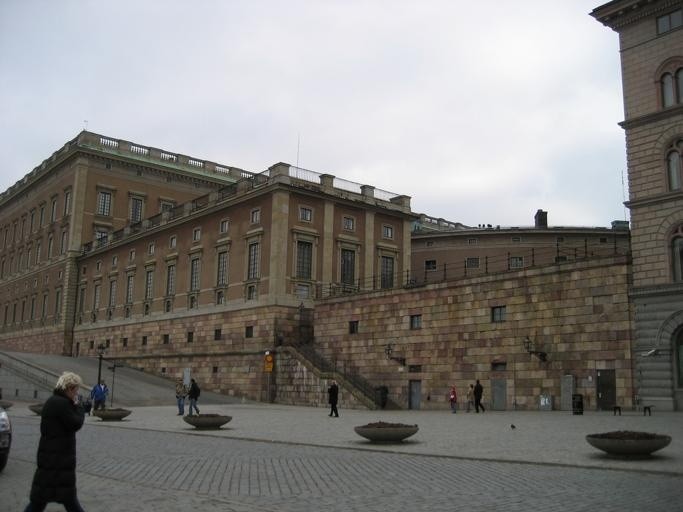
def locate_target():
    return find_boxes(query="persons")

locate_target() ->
[473,378,486,413]
[465,383,475,413]
[327,379,339,417]
[186,376,200,416]
[89,377,109,410]
[21,372,92,510]
[449,383,457,414]
[175,377,188,416]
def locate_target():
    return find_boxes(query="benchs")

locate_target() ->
[610,405,656,416]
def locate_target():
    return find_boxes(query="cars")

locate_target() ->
[0,403,12,471]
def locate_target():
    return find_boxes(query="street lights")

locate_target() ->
[96,342,105,384]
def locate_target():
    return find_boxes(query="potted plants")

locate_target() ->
[0,401,12,410]
[586,430,672,459]
[28,404,45,416]
[183,414,232,429]
[92,408,132,422]
[354,420,420,444]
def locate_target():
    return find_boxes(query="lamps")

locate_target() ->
[523,336,532,352]
[384,344,393,359]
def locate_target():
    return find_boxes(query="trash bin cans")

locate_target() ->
[376,386,387,409]
[572,394,583,415]
